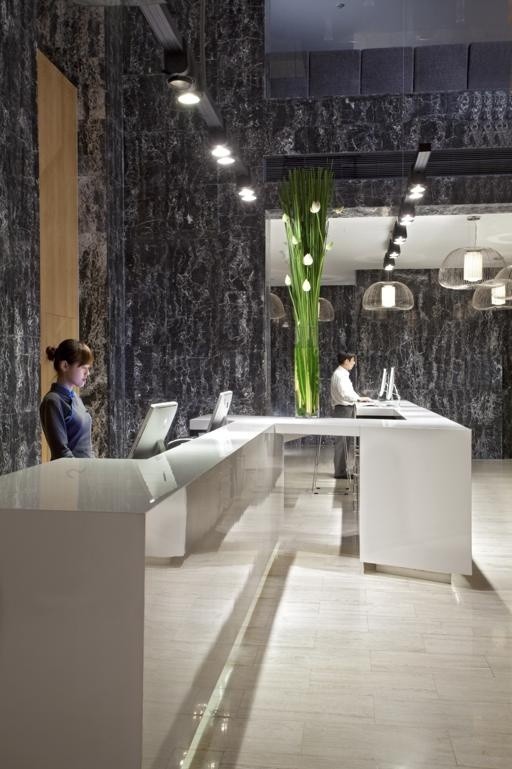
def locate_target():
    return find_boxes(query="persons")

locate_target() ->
[38,338,94,461]
[330,350,373,479]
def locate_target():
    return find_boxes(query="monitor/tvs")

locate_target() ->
[206,389,233,431]
[386,367,401,408]
[126,401,179,459]
[379,368,388,402]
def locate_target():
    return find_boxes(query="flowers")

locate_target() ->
[274,164,344,416]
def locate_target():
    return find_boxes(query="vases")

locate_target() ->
[294,326,320,418]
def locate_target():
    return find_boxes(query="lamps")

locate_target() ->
[383,174,427,271]
[210,142,236,165]
[437,216,509,291]
[239,186,258,202]
[472,265,512,312]
[361,271,415,311]
[318,297,335,322]
[167,74,200,107]
[270,292,287,320]
